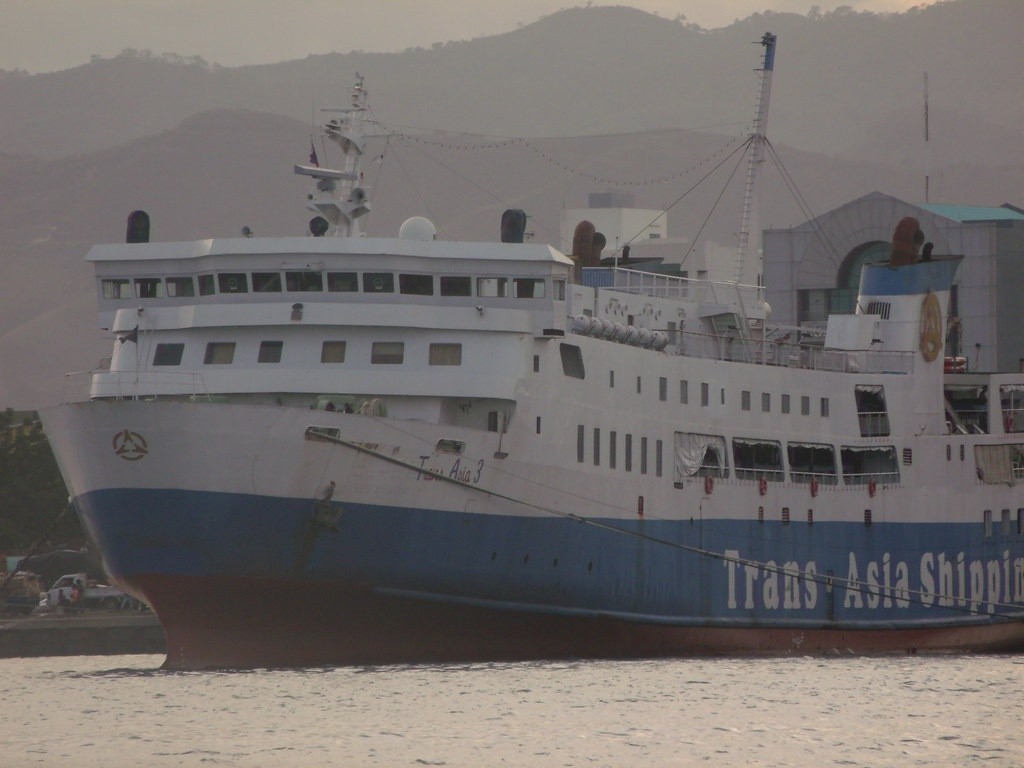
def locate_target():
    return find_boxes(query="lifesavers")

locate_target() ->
[943,356,967,374]
[1007,415,1014,431]
[868,478,877,497]
[704,475,714,494]
[760,477,768,496]
[811,478,819,497]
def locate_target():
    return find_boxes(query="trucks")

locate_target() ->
[46,573,125,612]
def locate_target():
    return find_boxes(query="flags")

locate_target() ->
[119,327,138,343]
[310,145,319,167]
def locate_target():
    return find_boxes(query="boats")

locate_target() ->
[34,30,1024,674]
